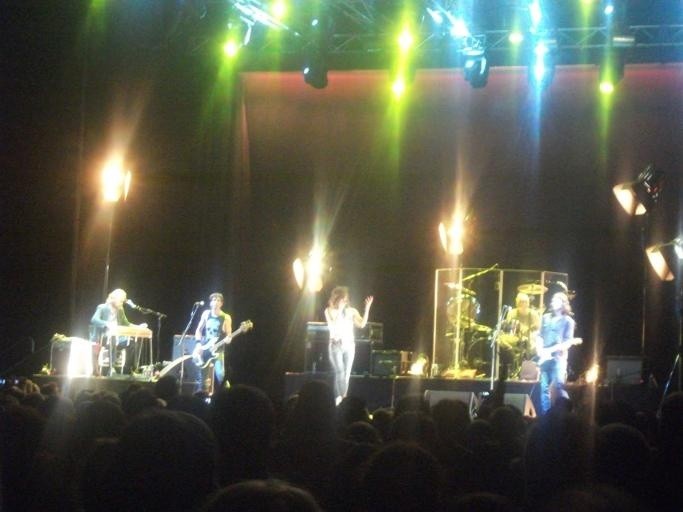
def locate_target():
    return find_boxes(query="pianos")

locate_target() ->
[107,325,152,338]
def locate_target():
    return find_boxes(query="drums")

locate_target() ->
[466,330,525,380]
[446,293,480,328]
[493,319,522,344]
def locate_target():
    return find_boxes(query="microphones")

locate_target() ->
[126,299,135,308]
[195,301,205,306]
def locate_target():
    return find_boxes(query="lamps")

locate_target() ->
[614,160,681,285]
[297,0,625,89]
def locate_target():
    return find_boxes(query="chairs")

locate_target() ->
[92,343,126,375]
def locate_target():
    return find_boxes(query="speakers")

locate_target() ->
[424,390,478,421]
[477,392,537,418]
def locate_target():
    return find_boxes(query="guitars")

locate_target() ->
[530,338,583,366]
[193,320,253,369]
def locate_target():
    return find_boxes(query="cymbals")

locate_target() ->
[517,284,548,294]
[444,282,476,296]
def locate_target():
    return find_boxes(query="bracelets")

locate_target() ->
[194,340,201,344]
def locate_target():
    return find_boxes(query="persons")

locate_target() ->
[505,290,542,339]
[529,291,575,418]
[1,368,682,512]
[89,286,149,376]
[323,285,373,409]
[192,291,234,394]
